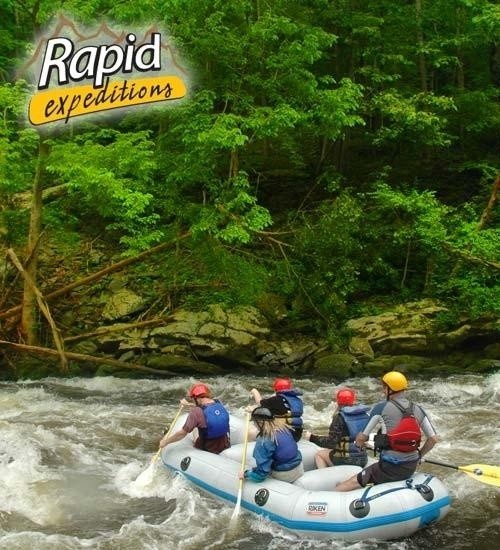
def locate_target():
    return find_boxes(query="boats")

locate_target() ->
[158,409,452,543]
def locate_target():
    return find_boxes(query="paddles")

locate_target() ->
[135,404,184,486]
[231,412,250,516]
[314,433,376,447]
[365,446,500,486]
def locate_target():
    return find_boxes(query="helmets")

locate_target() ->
[274,378,291,391]
[337,390,354,406]
[249,407,274,421]
[190,385,208,396]
[383,371,408,392]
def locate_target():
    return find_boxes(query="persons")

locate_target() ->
[239,377,304,483]
[305,372,437,492]
[159,385,231,454]
